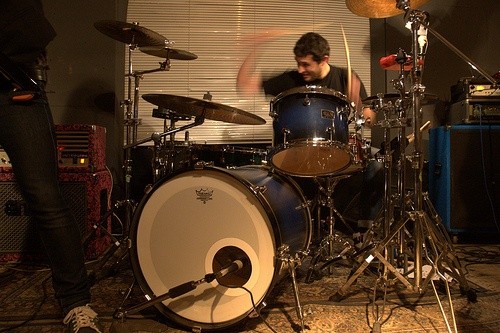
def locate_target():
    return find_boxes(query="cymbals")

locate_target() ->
[366,92,437,106]
[141,93,267,125]
[93,19,167,46]
[138,46,198,60]
[345,0,428,18]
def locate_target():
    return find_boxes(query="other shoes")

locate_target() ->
[63,304,102,333]
[353,228,381,263]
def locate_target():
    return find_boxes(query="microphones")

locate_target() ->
[215,257,247,279]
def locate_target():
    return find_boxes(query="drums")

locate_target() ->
[127,165,313,332]
[220,145,269,165]
[268,85,351,177]
[380,53,424,72]
[340,132,366,174]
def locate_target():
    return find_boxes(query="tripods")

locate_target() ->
[309,0,497,333]
[82,37,139,251]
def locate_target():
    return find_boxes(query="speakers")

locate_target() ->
[0,169,112,267]
[427,125,500,233]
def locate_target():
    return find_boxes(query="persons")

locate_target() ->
[235,32,387,254]
[0,0,103,333]
